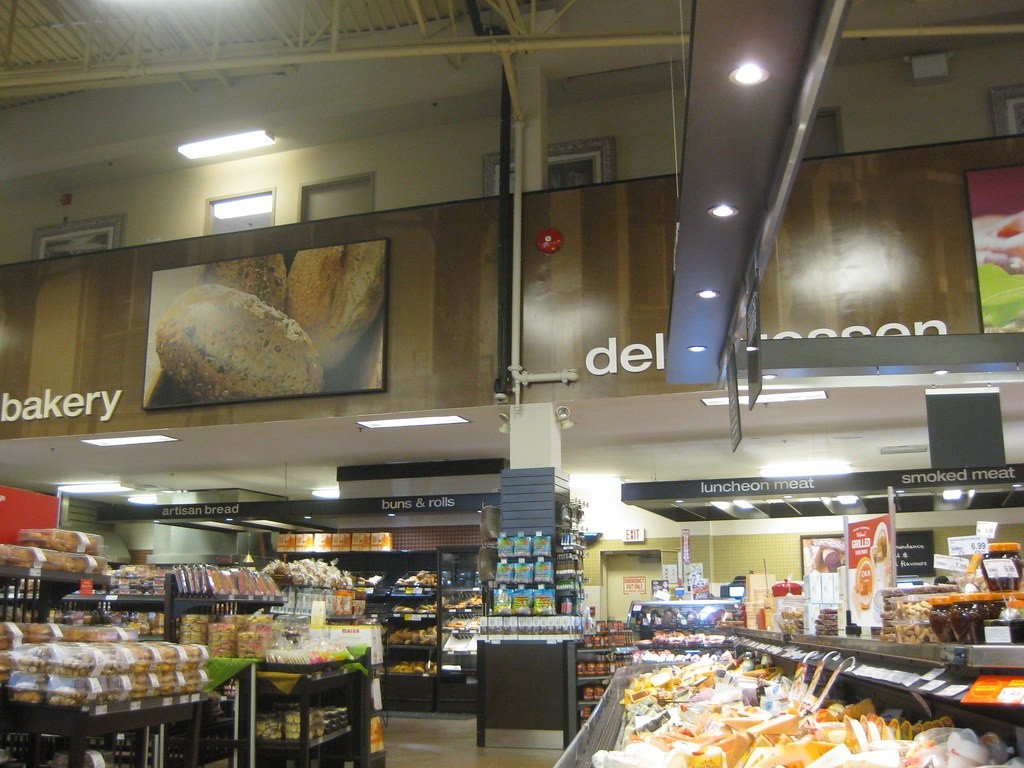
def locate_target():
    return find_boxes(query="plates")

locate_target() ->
[873,521,888,548]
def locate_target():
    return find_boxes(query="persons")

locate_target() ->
[934,575,949,585]
[652,581,669,595]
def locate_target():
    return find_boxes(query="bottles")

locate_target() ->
[929,542,1024,643]
[740,651,773,673]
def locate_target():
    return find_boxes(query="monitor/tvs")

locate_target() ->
[728,585,745,599]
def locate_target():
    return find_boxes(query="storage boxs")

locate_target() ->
[332,534,351,552]
[314,533,332,552]
[746,573,776,604]
[296,534,314,553]
[391,600,426,616]
[350,570,390,588]
[278,534,295,552]
[371,533,393,552]
[744,601,776,631]
[214,554,240,563]
[397,571,450,590]
[351,533,370,551]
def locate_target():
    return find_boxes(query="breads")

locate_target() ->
[349,570,483,673]
[157,242,386,402]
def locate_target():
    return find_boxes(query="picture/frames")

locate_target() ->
[31,212,126,266]
[964,163,1024,340]
[137,241,389,412]
[800,534,845,581]
[480,136,615,203]
[988,82,1024,137]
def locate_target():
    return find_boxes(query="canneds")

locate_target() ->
[576,654,633,675]
[583,619,624,647]
[580,706,591,728]
[582,684,605,702]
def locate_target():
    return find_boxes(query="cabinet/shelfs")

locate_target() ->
[0,545,483,768]
[575,602,744,730]
